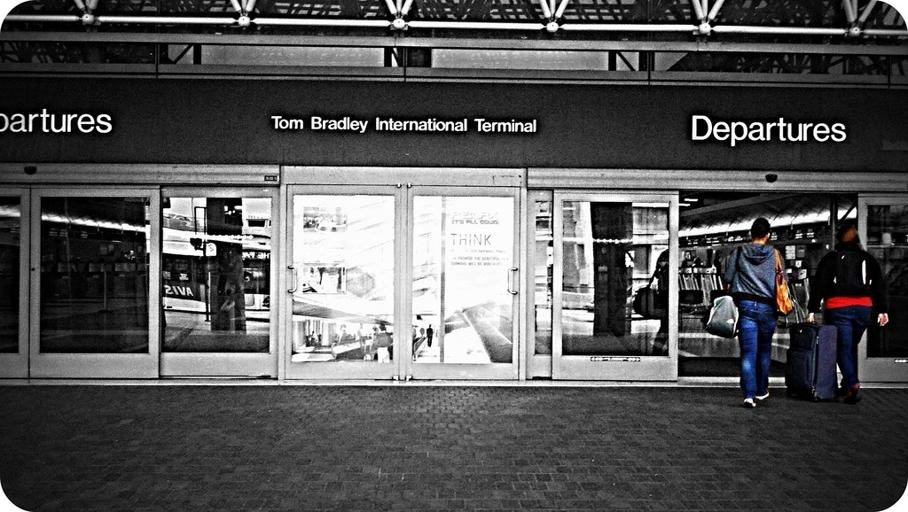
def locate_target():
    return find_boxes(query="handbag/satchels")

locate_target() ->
[702,294,740,340]
[632,268,665,320]
[773,248,795,316]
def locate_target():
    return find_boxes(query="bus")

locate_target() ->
[145,227,270,321]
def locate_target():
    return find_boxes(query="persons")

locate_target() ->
[650,238,669,354]
[545,239,554,290]
[305,322,434,362]
[807,223,891,404]
[681,246,735,291]
[721,215,789,408]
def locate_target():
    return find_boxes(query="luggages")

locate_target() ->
[785,321,839,401]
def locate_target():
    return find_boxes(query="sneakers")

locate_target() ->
[840,386,862,405]
[743,393,770,408]
